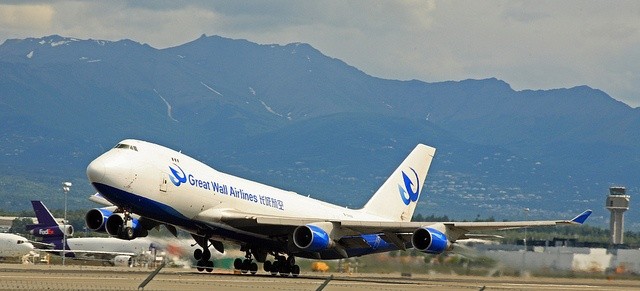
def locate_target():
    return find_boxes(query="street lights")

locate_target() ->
[59,180,73,267]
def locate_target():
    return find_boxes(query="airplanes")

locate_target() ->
[30,199,227,273]
[85,138,593,278]
[0,231,37,267]
